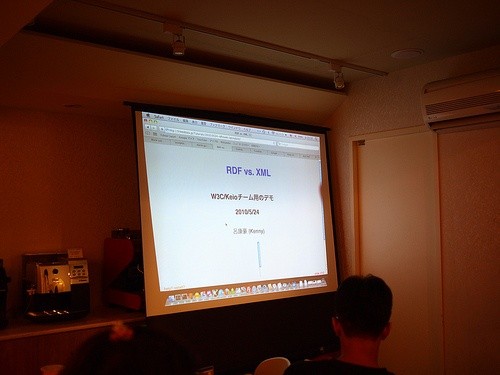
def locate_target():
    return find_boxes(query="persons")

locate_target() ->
[283,273,393,375]
[58,318,196,375]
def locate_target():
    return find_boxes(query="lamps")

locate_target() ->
[172,33,186,56]
[334,72,345,89]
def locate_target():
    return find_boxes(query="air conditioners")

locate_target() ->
[422,68,500,134]
